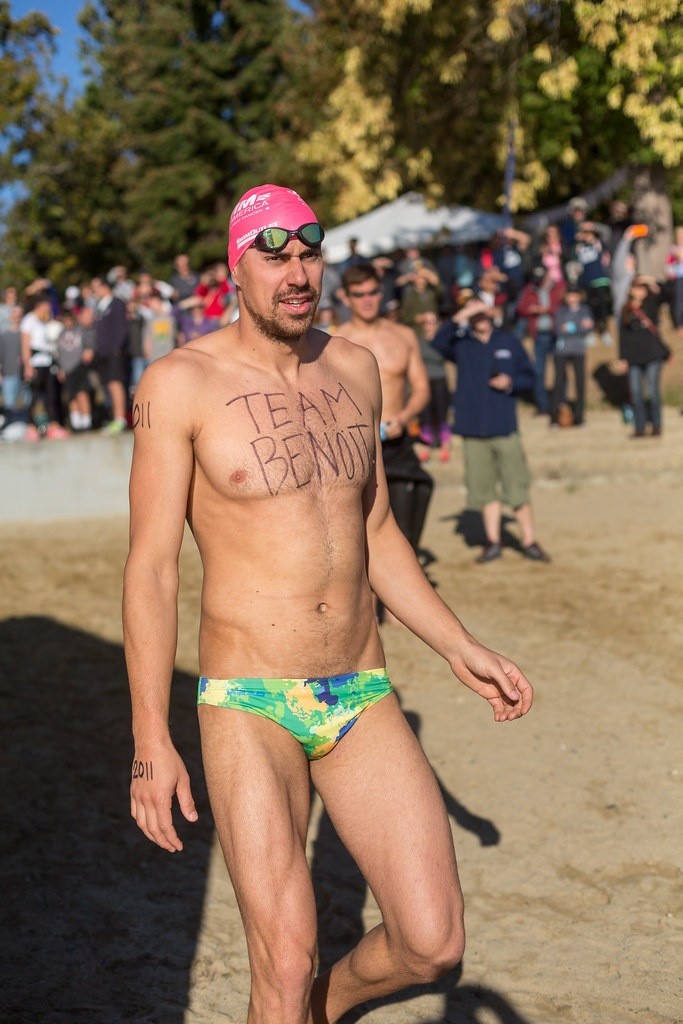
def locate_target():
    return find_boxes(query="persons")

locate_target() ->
[119,182,536,1024]
[2,182,683,630]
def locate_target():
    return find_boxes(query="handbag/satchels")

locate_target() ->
[661,342,671,361]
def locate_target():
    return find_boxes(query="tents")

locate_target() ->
[310,189,518,268]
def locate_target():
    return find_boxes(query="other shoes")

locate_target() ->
[46,422,68,440]
[102,419,126,437]
[476,543,503,563]
[418,451,430,463]
[522,542,549,563]
[26,423,39,443]
[440,449,448,462]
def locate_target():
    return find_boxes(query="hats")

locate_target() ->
[568,198,587,214]
[228,185,318,273]
[531,265,547,281]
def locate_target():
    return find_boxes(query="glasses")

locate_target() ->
[249,222,325,252]
[349,288,381,299]
